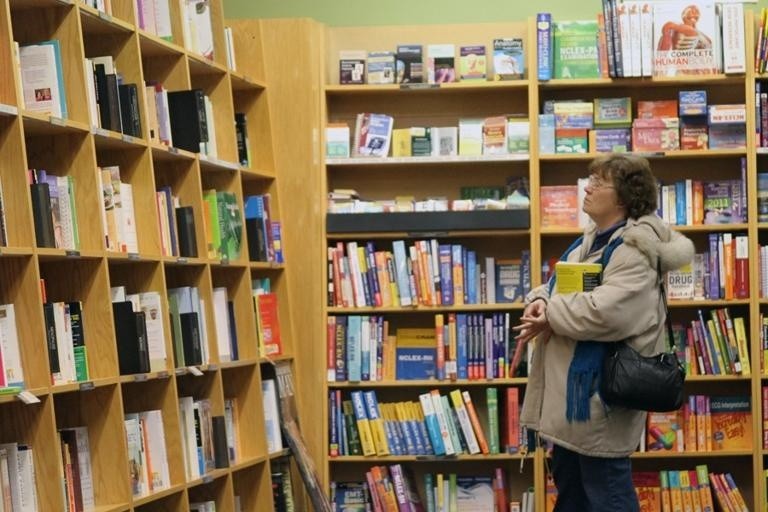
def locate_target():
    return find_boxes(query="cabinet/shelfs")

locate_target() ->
[252,17,768,512]
[1,2,301,512]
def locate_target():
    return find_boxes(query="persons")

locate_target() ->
[510,153,696,512]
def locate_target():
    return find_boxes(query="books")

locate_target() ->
[753,6,768,512]
[328,176,531,215]
[0,0,295,512]
[338,37,526,84]
[537,0,747,80]
[541,177,590,230]
[640,157,755,452]
[546,473,559,512]
[326,240,532,512]
[634,464,749,512]
[327,111,530,159]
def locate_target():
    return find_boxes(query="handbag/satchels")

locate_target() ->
[599,342,684,412]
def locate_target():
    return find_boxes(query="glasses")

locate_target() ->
[588,174,615,189]
